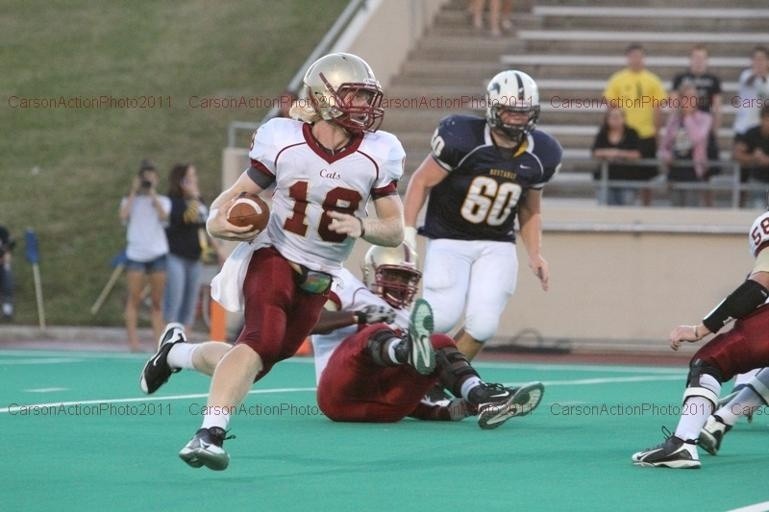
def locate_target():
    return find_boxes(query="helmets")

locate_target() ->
[302,53,384,134]
[486,69,540,139]
[362,243,423,310]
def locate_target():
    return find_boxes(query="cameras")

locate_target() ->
[140,180,151,190]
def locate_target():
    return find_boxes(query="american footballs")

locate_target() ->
[226,195,270,230]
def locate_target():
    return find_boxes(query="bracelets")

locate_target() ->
[694,324,702,340]
[352,214,366,238]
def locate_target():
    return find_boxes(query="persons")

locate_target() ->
[593,45,769,208]
[0,224,15,322]
[402,69,563,401]
[629,212,769,468]
[304,236,544,431]
[164,164,209,343]
[138,52,406,472]
[466,0,506,39]
[119,159,171,352]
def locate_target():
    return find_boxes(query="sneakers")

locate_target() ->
[140,322,186,394]
[698,413,733,456]
[179,428,235,470]
[404,300,437,374]
[633,425,699,468]
[448,398,477,422]
[478,381,545,429]
